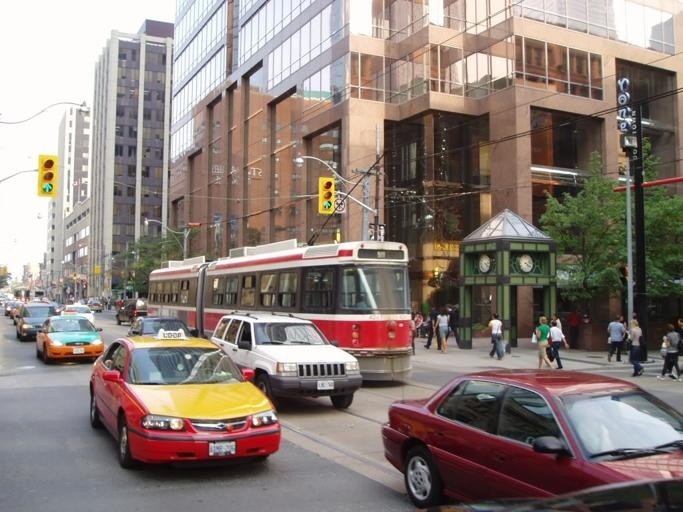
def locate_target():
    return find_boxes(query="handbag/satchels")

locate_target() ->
[492,337,496,343]
[545,347,554,362]
[548,336,552,344]
[448,326,452,332]
[531,332,537,343]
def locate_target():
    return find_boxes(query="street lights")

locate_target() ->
[619,133,647,360]
[142,217,192,262]
[291,154,370,244]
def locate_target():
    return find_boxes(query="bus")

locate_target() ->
[146,237,413,384]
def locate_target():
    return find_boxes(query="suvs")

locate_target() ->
[209,308,363,411]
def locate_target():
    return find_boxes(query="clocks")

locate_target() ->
[518,254,533,274]
[477,254,491,274]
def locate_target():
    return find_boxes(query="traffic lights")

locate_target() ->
[37,154,60,198]
[317,177,334,216]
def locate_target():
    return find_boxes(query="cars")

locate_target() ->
[59,305,94,325]
[34,310,103,364]
[0,287,61,325]
[14,303,59,341]
[86,298,103,313]
[124,315,195,340]
[418,477,681,511]
[378,366,681,512]
[85,326,281,470]
[113,297,147,326]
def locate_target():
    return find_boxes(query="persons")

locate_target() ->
[101,297,111,310]
[413,303,683,382]
[353,295,367,314]
